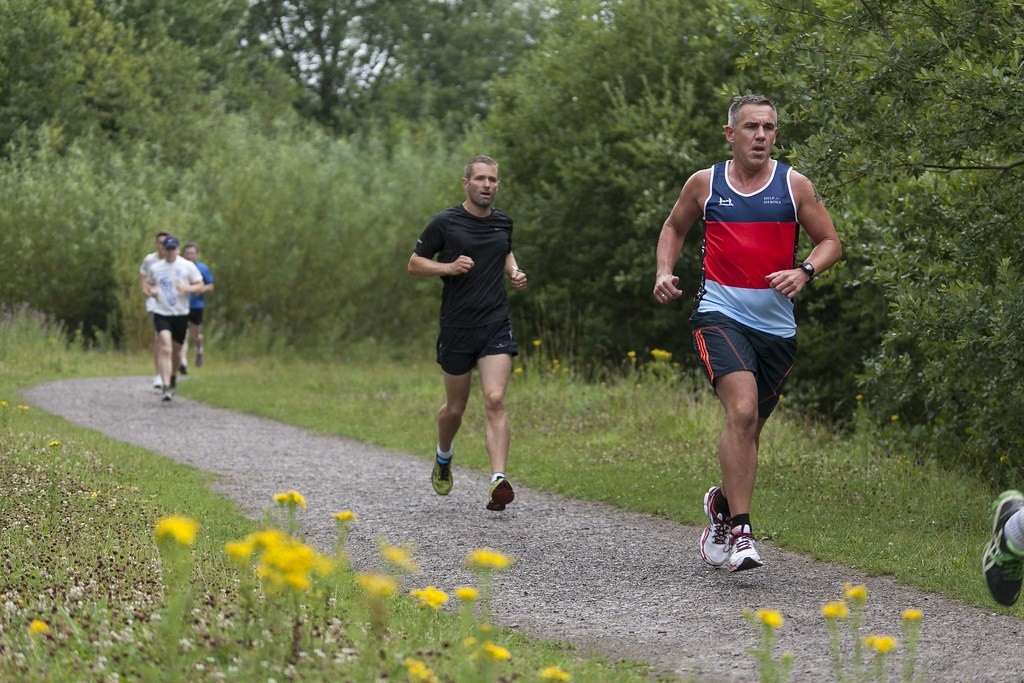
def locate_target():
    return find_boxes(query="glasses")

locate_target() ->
[166,246,176,250]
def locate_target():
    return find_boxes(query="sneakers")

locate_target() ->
[722,524,763,572]
[983,489,1024,607]
[700,486,730,566]
[431,447,454,495]
[485,477,514,512]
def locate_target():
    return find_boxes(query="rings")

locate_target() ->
[661,294,665,298]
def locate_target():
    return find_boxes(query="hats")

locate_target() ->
[162,237,179,247]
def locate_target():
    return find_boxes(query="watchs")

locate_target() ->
[800,262,814,279]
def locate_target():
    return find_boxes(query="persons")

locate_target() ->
[179,244,215,375]
[146,236,203,401]
[140,232,169,387]
[407,155,527,512]
[980,489,1024,608]
[653,95,841,573]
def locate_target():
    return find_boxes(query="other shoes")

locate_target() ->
[162,391,173,402]
[196,348,203,366]
[179,365,187,375]
[170,377,175,389]
[153,375,162,388]
